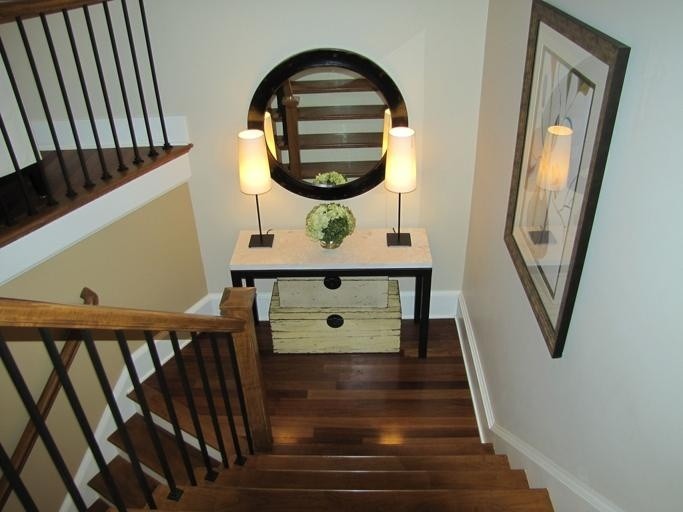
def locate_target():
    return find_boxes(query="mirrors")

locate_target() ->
[246,47,409,200]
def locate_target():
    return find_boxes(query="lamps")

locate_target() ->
[263,111,276,161]
[381,107,392,159]
[237,129,275,248]
[528,126,576,247]
[384,126,416,248]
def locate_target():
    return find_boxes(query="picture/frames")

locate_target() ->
[502,0,631,360]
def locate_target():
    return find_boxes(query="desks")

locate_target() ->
[228,228,432,359]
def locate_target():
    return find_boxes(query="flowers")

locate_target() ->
[304,201,356,249]
[312,170,347,188]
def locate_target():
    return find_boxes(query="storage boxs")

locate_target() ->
[268,276,402,353]
